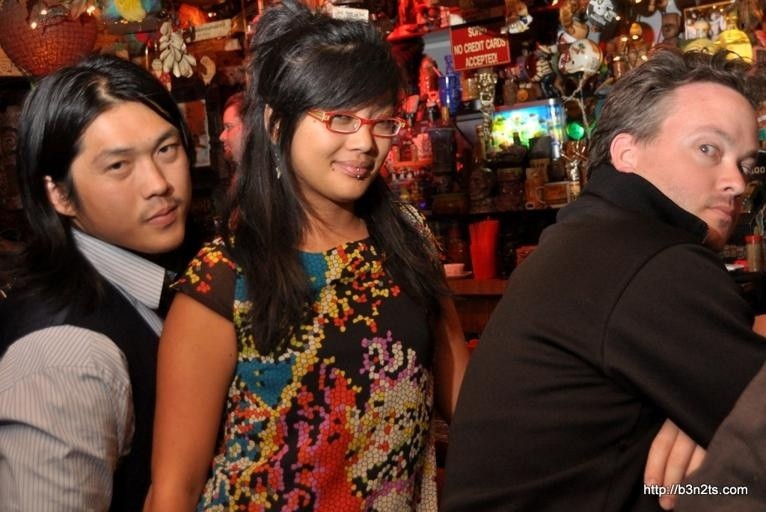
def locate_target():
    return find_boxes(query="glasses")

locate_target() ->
[306,110,407,138]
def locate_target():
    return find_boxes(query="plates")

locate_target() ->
[446,270,473,279]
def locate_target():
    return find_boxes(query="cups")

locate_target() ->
[444,263,465,274]
[535,181,571,212]
[438,53,463,119]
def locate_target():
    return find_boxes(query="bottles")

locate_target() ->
[545,140,568,182]
[745,233,766,276]
[144,18,173,94]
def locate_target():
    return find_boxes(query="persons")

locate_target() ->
[140,2,469,512]
[217,92,248,235]
[667,361,766,511]
[0,50,206,512]
[440,46,766,511]
